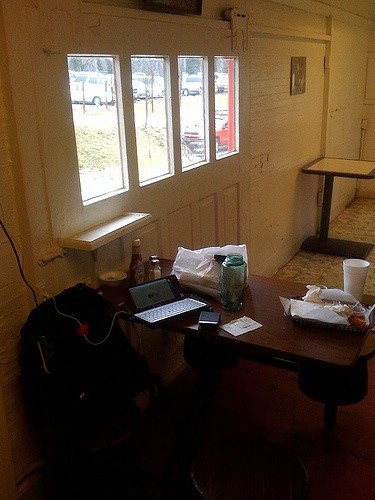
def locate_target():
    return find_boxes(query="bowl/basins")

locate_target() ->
[98,270,127,287]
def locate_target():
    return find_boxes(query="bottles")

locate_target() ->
[146,255,161,282]
[219,253,248,312]
[128,239,145,288]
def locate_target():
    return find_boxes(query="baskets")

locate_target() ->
[283,296,375,333]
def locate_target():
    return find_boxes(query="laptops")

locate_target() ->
[125,274,213,330]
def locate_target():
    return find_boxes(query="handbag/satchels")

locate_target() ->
[168,245,249,303]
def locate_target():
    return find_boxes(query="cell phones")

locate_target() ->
[198,311,221,325]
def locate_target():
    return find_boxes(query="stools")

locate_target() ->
[298,332,375,406]
[190,436,308,500]
[80,415,136,453]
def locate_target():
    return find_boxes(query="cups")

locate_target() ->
[342,258,370,304]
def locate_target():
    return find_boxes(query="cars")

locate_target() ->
[181,114,230,151]
[68,71,229,105]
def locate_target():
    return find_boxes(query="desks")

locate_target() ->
[93,257,375,426]
[300,157,375,260]
